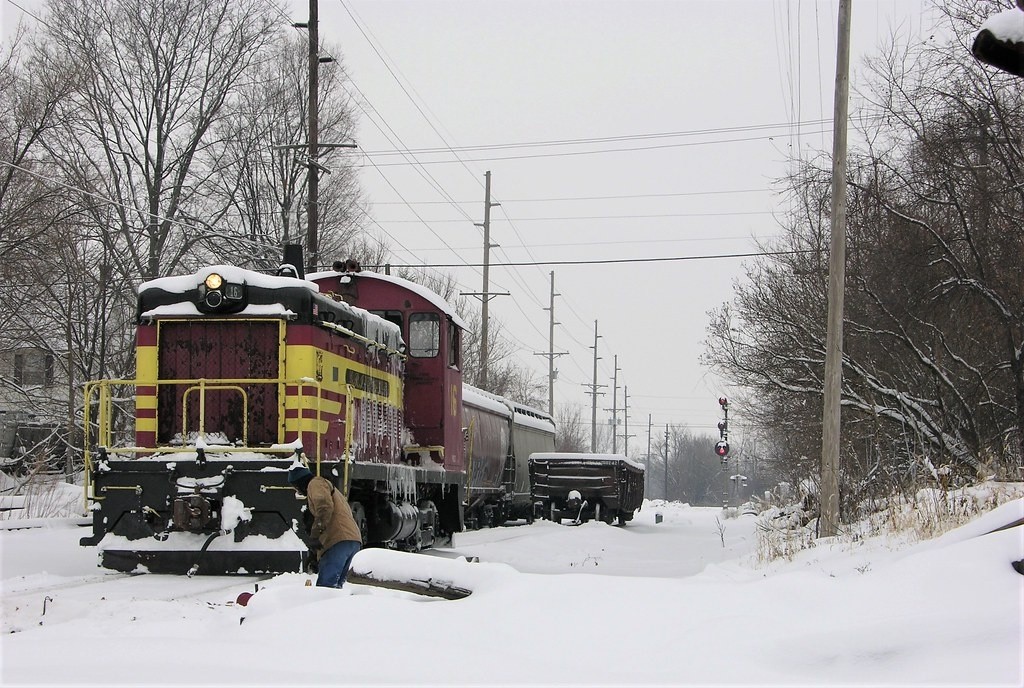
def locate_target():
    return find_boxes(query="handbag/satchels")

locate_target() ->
[304,508,315,536]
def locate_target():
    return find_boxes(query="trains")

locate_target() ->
[91,255,557,579]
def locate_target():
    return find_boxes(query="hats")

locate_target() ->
[288,460,311,482]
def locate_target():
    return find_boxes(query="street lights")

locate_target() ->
[719,397,726,406]
[715,441,730,455]
[718,421,726,430]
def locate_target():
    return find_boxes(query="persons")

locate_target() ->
[287,466,363,587]
